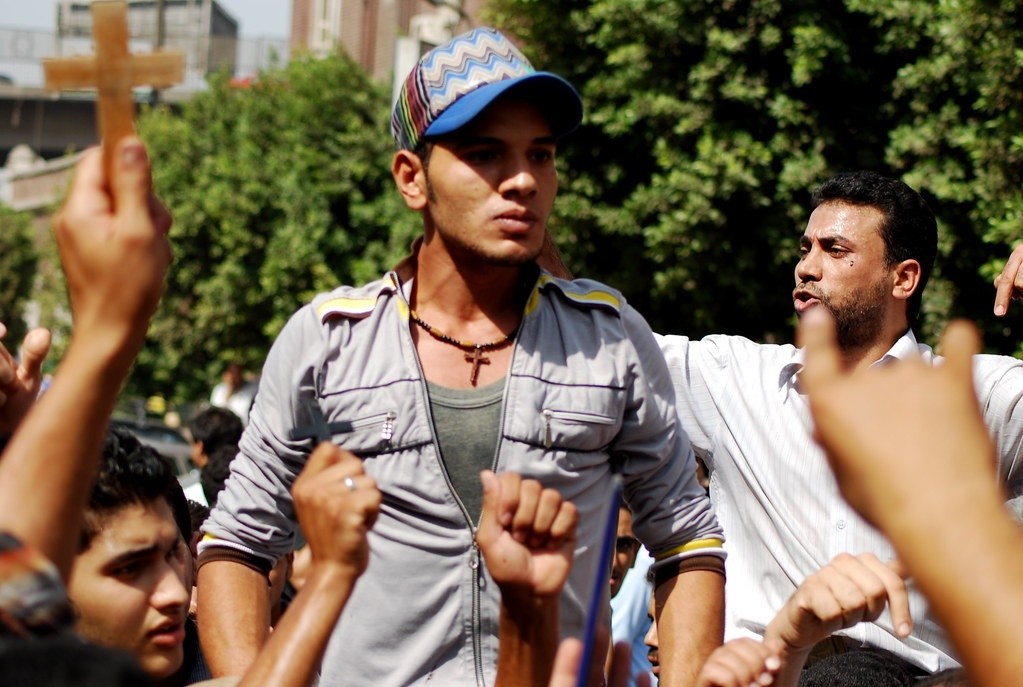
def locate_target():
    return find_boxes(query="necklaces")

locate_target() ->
[408,308,522,383]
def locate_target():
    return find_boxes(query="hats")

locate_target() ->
[392,27,584,153]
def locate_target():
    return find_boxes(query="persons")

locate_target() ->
[0,135,177,687]
[146,352,710,687]
[533,168,1023,687]
[195,24,729,687]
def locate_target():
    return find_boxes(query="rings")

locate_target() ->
[344,476,356,491]
